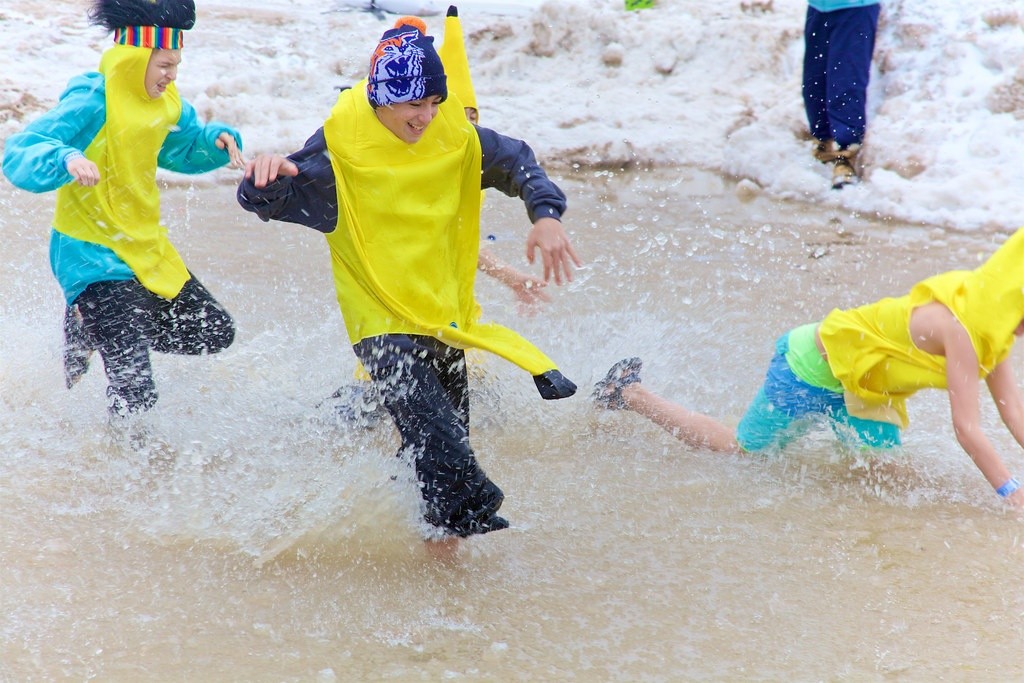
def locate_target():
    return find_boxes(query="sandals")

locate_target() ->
[590,356,642,413]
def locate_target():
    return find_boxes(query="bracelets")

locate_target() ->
[995,476,1021,497]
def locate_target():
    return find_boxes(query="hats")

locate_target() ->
[368,17,448,109]
[434,5,479,115]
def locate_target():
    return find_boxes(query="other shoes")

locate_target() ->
[64,303,95,390]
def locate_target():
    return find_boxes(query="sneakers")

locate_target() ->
[833,144,866,188]
[811,140,845,163]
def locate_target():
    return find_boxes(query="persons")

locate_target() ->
[800,0,880,190]
[0,0,247,423]
[593,226,1022,510]
[314,5,552,432]
[236,17,583,558]
